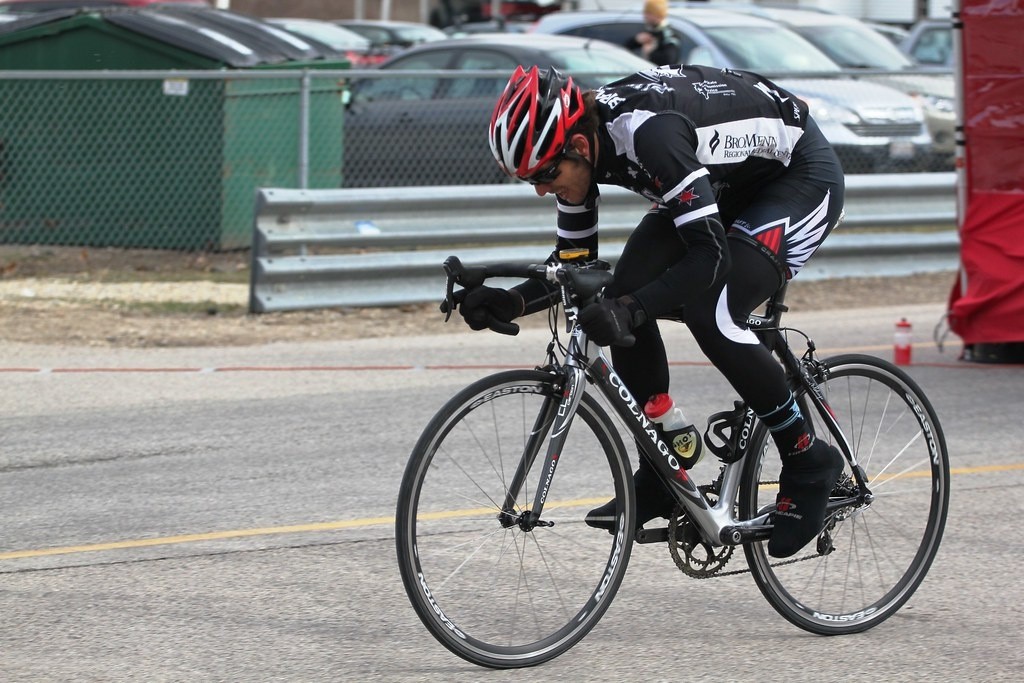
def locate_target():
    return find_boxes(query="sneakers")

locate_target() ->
[766,444,845,558]
[585,459,695,528]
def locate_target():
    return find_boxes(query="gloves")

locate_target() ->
[439,289,525,334]
[577,296,649,347]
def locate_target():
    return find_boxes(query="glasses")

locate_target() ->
[520,127,573,184]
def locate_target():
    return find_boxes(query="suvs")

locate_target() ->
[529,11,936,175]
[716,6,956,170]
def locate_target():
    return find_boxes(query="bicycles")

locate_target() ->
[395,248,952,672]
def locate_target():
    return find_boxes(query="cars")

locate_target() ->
[262,16,451,64]
[343,32,660,188]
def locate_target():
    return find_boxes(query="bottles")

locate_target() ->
[644,392,707,470]
[892,317,913,366]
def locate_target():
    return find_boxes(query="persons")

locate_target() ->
[440,64,845,559]
[622,0,682,66]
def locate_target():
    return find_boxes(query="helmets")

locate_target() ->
[487,64,587,179]
